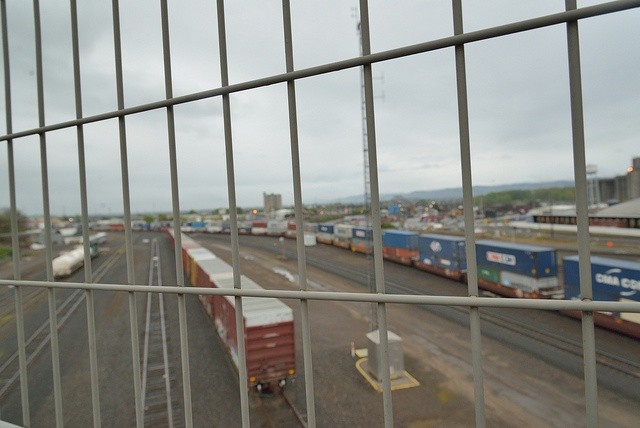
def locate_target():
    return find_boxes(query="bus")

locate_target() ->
[51,247,100,277]
[79,232,108,247]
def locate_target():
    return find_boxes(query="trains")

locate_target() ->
[166,224,296,394]
[90,220,639,346]
